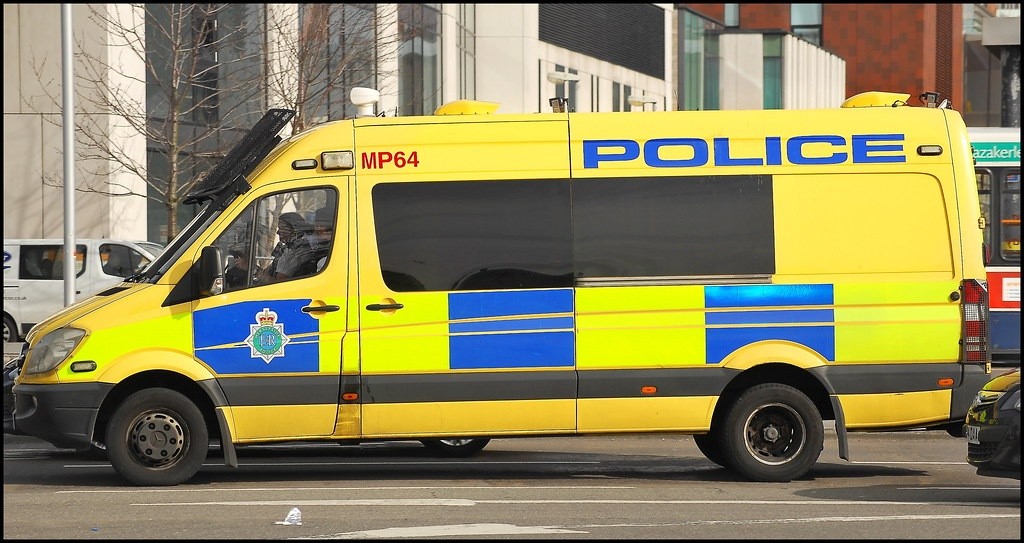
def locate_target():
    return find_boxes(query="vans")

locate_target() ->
[4,237,173,346]
[13,87,988,487]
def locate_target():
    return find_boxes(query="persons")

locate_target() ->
[235,207,334,283]
